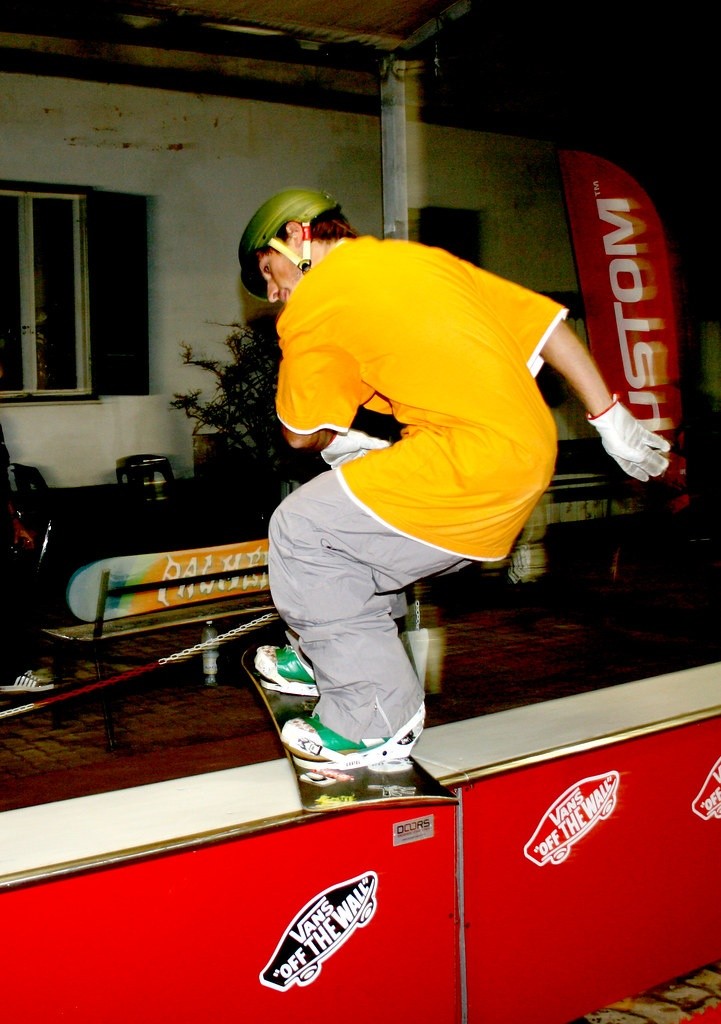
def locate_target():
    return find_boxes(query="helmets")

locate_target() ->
[237,184,338,299]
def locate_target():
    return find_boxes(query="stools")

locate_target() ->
[113,455,176,501]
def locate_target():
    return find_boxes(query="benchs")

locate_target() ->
[41,538,274,750]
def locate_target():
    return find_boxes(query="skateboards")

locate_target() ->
[240,645,460,817]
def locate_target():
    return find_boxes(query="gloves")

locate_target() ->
[319,427,393,468]
[585,393,671,482]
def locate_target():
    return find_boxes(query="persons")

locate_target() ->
[239,187,673,761]
[0,335,55,695]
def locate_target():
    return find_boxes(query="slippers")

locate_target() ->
[0,670,54,692]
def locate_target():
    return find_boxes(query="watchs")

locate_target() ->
[9,508,25,521]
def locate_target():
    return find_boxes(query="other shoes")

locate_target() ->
[280,702,427,769]
[253,644,322,698]
